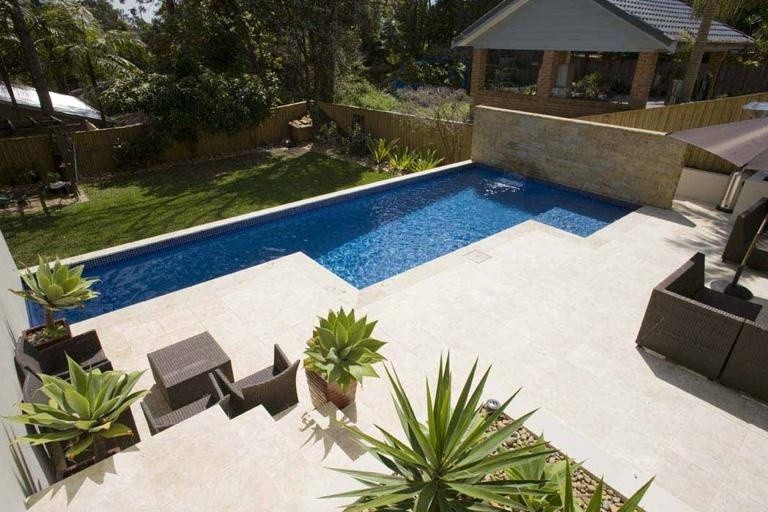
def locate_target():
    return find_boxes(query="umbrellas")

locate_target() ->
[666,115,768,302]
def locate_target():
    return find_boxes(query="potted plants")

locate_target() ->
[302,306,388,411]
[3,351,152,480]
[6,253,103,349]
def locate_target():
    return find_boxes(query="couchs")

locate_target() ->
[721,196,768,273]
[635,253,767,404]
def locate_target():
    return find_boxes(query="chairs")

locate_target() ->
[21,371,141,451]
[14,329,107,375]
[214,343,301,415]
[140,371,231,435]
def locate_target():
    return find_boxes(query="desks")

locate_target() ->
[147,330,236,410]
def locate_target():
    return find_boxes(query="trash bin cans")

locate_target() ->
[716,172,748,213]
